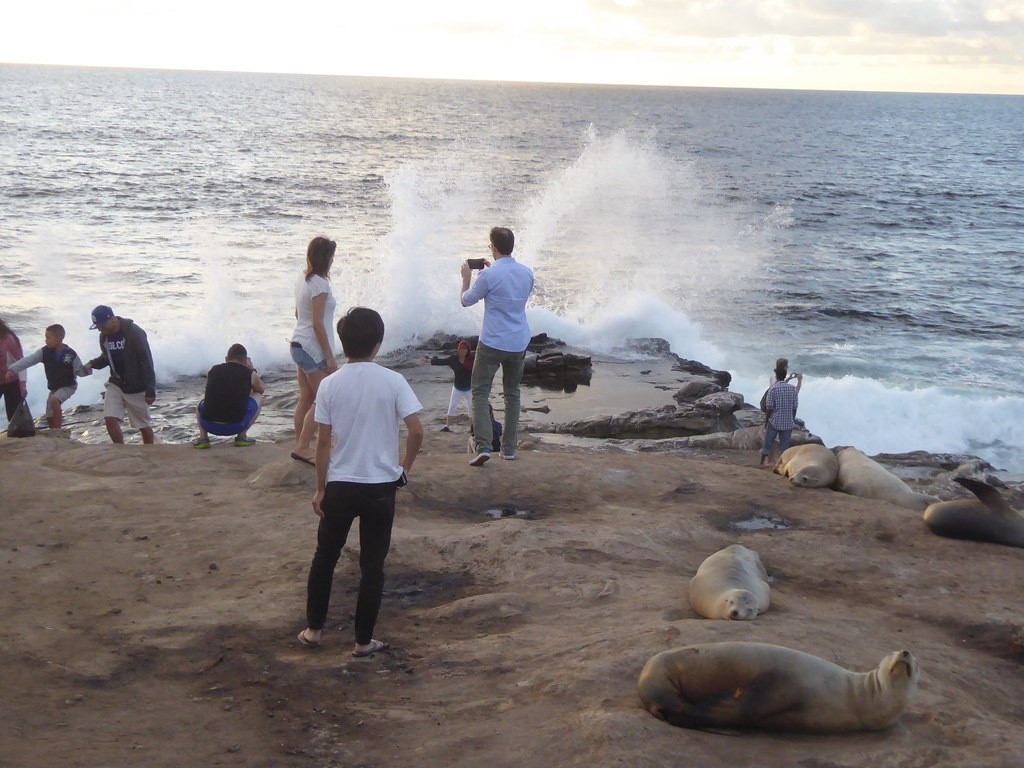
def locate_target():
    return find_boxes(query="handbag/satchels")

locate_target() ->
[7,400,35,437]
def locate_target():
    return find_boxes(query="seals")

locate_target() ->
[636,639,922,738]
[685,542,773,621]
[921,476,1024,551]
[770,443,945,512]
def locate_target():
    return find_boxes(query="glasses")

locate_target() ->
[489,243,492,249]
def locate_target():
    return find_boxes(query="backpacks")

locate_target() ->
[760,388,769,412]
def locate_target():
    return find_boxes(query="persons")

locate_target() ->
[0,320,28,423]
[84,305,156,444]
[417,340,475,433]
[460,227,534,466]
[298,307,424,657]
[760,359,803,464]
[5,324,88,429]
[193,344,264,449]
[290,238,337,467]
[468,404,502,454]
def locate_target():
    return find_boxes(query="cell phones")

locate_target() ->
[468,259,484,269]
[795,374,799,377]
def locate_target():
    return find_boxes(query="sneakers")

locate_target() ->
[192,438,210,448]
[235,436,255,446]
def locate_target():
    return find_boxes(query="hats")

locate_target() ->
[89,305,112,330]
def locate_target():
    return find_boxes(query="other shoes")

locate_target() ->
[499,452,514,460]
[469,448,490,465]
[439,427,449,431]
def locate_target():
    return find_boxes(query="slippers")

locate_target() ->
[291,452,315,467]
[298,630,321,647]
[352,639,388,656]
